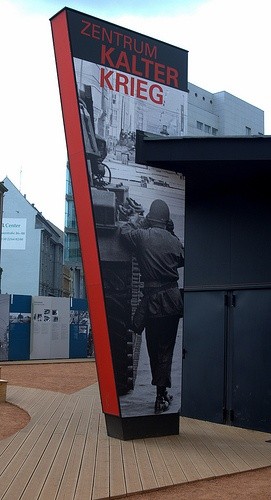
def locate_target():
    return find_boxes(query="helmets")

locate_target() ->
[146,199,170,224]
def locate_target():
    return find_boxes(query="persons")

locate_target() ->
[118,198,185,412]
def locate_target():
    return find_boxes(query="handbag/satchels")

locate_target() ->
[133,295,149,335]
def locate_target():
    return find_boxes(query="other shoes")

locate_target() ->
[154,393,174,413]
